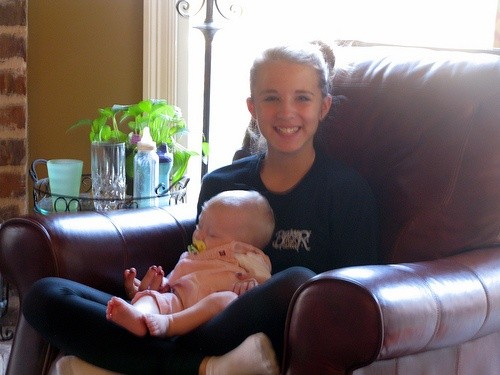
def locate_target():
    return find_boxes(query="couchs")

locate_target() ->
[0,37,500,374]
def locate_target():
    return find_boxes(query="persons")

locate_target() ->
[20,41,387,375]
[104,189,276,338]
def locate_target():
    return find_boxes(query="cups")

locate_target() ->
[47,159,83,210]
[91,140,126,209]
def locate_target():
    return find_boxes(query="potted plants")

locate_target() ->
[64,99,210,196]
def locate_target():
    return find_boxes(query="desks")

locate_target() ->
[29,159,190,216]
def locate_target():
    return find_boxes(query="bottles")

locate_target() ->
[158,144,173,194]
[134,127,159,206]
